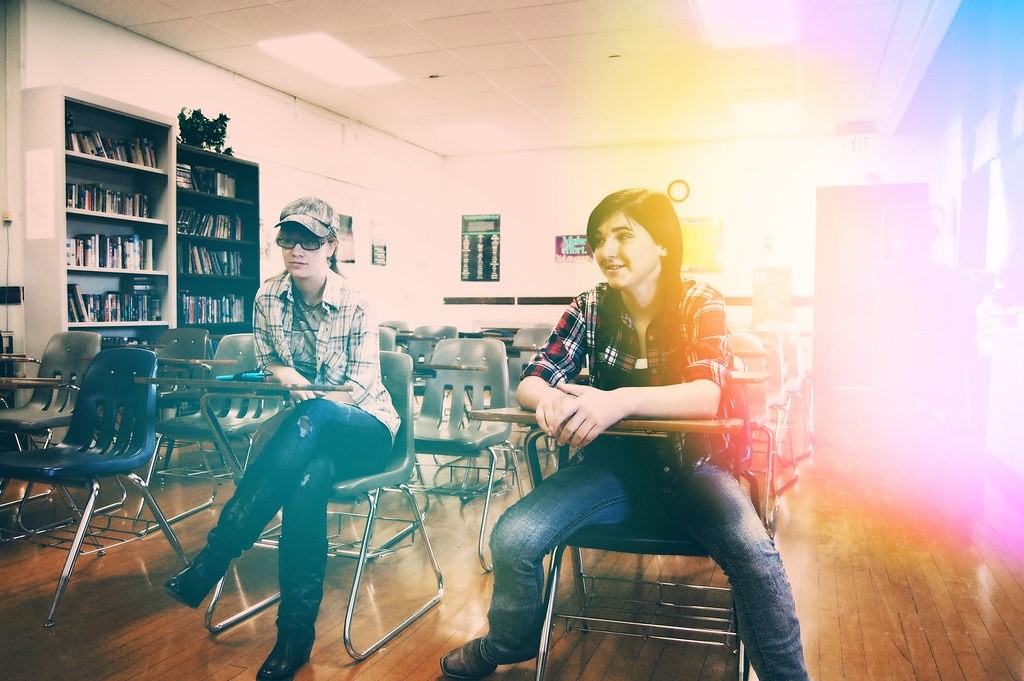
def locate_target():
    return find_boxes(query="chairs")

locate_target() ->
[509,327,555,486]
[414,338,526,575]
[203,351,444,661]
[154,328,210,486]
[133,334,285,537]
[0,347,191,627]
[0,331,128,543]
[379,326,397,351]
[408,325,459,421]
[380,321,410,353]
[532,322,817,681]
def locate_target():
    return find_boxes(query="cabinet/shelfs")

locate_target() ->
[23,84,177,401]
[177,142,261,335]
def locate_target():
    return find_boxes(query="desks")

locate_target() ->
[0,353,29,363]
[506,344,541,351]
[731,349,770,360]
[403,335,446,342]
[730,370,771,412]
[470,407,746,488]
[416,360,490,377]
[481,327,520,331]
[130,375,356,475]
[0,377,64,389]
[159,356,239,366]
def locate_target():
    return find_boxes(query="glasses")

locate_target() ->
[276,231,332,251]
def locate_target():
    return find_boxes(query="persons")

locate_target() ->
[161,198,403,681]
[441,190,812,681]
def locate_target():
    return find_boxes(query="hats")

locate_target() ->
[274,196,340,237]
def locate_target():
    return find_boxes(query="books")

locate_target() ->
[176,156,251,325]
[54,122,172,329]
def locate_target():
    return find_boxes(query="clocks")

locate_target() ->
[668,180,690,203]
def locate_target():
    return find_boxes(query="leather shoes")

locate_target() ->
[440,638,498,679]
[161,544,231,609]
[256,624,315,681]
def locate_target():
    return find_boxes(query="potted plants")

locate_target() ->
[178,107,231,153]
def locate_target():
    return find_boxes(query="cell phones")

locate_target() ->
[216,372,269,382]
